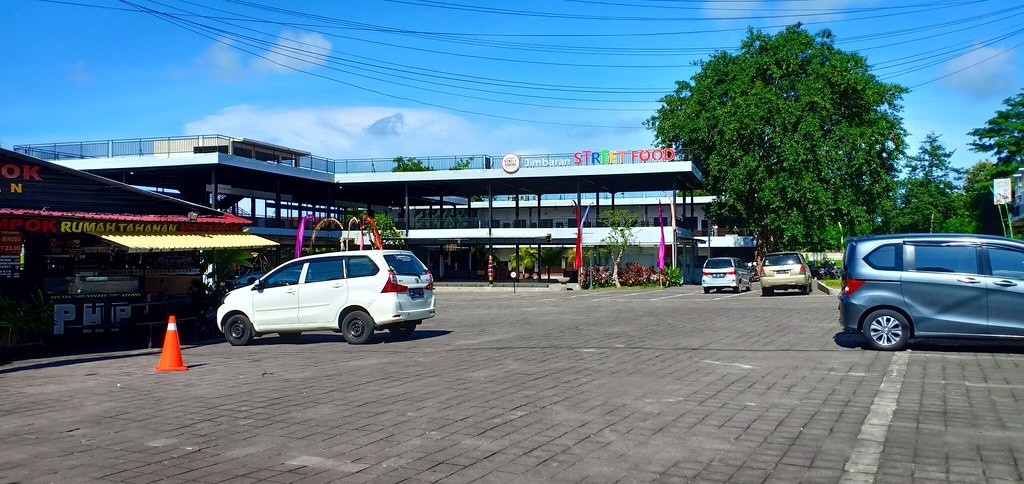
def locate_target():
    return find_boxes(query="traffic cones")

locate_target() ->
[152,313,189,372]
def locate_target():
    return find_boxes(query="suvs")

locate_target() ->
[759,251,813,296]
[216,249,436,347]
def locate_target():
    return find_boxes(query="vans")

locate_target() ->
[838,233,1024,351]
[702,257,752,293]
